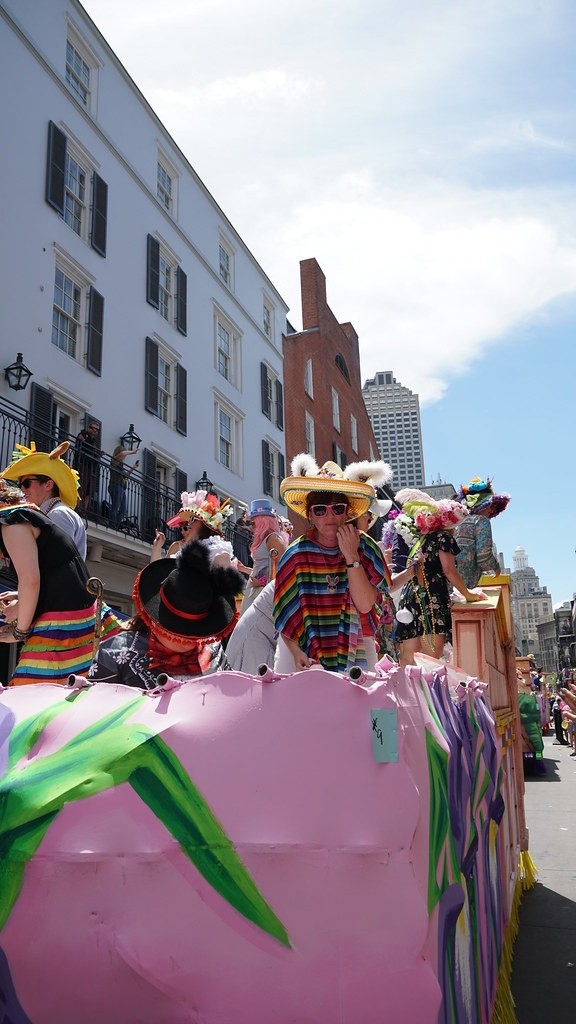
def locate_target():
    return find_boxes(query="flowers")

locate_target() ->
[180,490,234,538]
[456,478,494,510]
[382,489,470,549]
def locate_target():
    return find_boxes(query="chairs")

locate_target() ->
[102,499,140,538]
[145,516,179,549]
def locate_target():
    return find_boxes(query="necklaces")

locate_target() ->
[408,563,435,653]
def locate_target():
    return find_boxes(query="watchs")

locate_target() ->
[346,560,362,568]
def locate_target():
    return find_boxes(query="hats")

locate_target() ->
[1,478,41,512]
[365,496,392,530]
[279,453,392,523]
[249,499,277,518]
[449,476,510,520]
[395,488,469,534]
[2,441,82,511]
[166,490,233,535]
[133,534,246,645]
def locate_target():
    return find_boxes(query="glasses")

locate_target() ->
[188,516,200,523]
[310,503,347,518]
[179,526,189,532]
[89,425,99,432]
[251,521,256,527]
[18,478,42,489]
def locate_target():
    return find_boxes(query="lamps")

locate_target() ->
[89,545,103,562]
[3,353,33,390]
[195,471,214,493]
[120,424,141,453]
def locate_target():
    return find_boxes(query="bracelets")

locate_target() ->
[13,627,29,640]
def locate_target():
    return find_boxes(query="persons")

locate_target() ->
[238,499,285,616]
[108,446,140,530]
[550,684,576,753]
[74,421,100,517]
[225,578,279,676]
[390,488,484,667]
[87,539,245,694]
[150,490,234,569]
[0,441,87,562]
[446,477,510,594]
[0,477,122,686]
[234,510,252,534]
[273,454,393,673]
